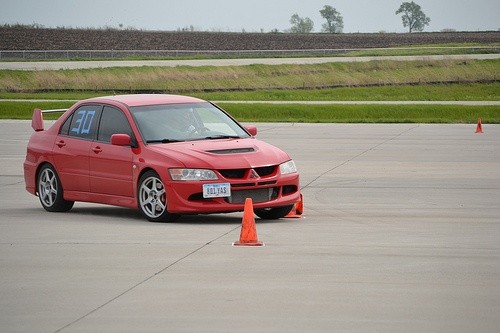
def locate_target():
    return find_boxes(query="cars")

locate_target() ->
[23,93,301,222]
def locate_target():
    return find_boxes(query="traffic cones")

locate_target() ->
[295,193,304,215]
[232,197,263,246]
[283,203,301,218]
[474,118,484,133]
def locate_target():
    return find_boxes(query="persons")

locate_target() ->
[164,108,199,140]
